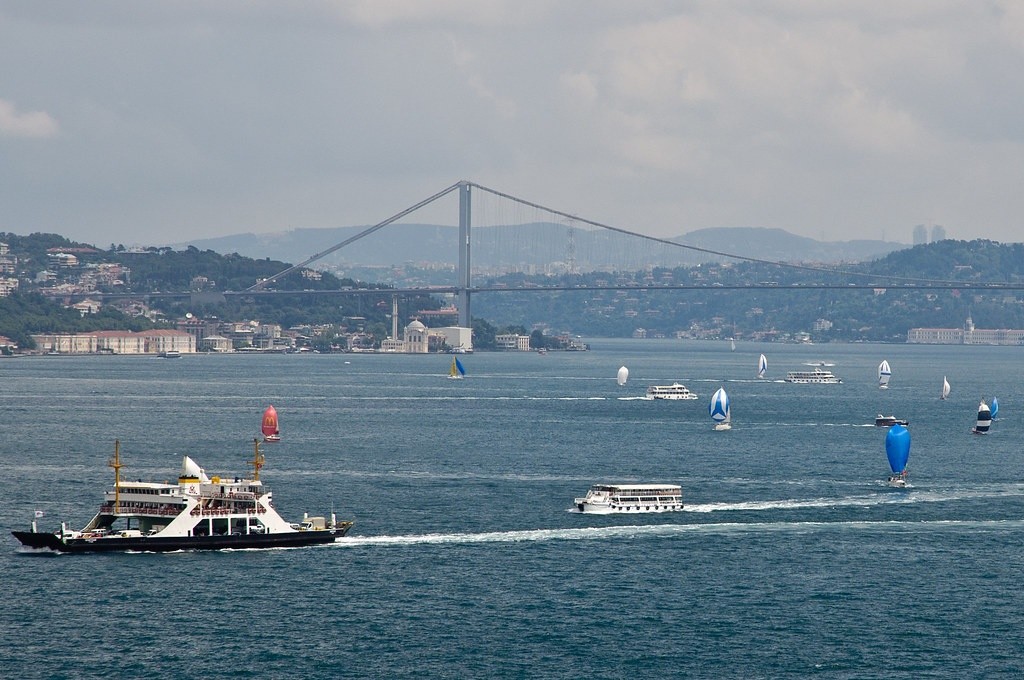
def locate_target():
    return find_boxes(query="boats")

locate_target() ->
[646,382,698,400]
[783,366,845,384]
[572,484,683,515]
[156,349,182,359]
[807,361,836,367]
[875,414,909,429]
[9,434,354,556]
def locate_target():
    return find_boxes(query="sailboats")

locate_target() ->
[886,423,911,488]
[988,395,999,421]
[446,355,466,380]
[262,404,281,442]
[940,375,951,401]
[756,353,768,380]
[877,359,892,390]
[707,385,732,431]
[730,340,736,352]
[617,365,629,387]
[971,397,992,435]
